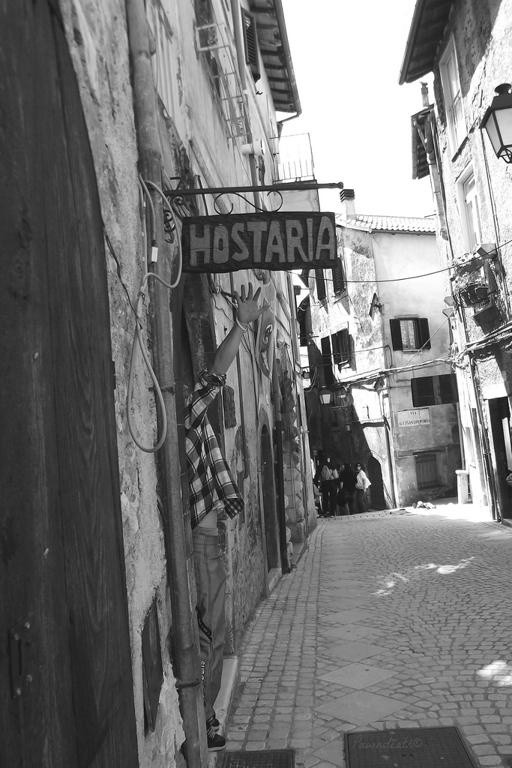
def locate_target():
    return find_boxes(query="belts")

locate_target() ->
[192,525,219,537]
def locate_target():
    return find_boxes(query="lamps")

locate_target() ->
[479,84,511,164]
[318,385,334,407]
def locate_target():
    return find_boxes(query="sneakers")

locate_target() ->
[206,714,226,751]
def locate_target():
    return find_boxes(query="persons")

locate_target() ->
[183,282,270,751]
[313,462,372,519]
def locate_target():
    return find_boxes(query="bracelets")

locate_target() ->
[236,319,247,331]
